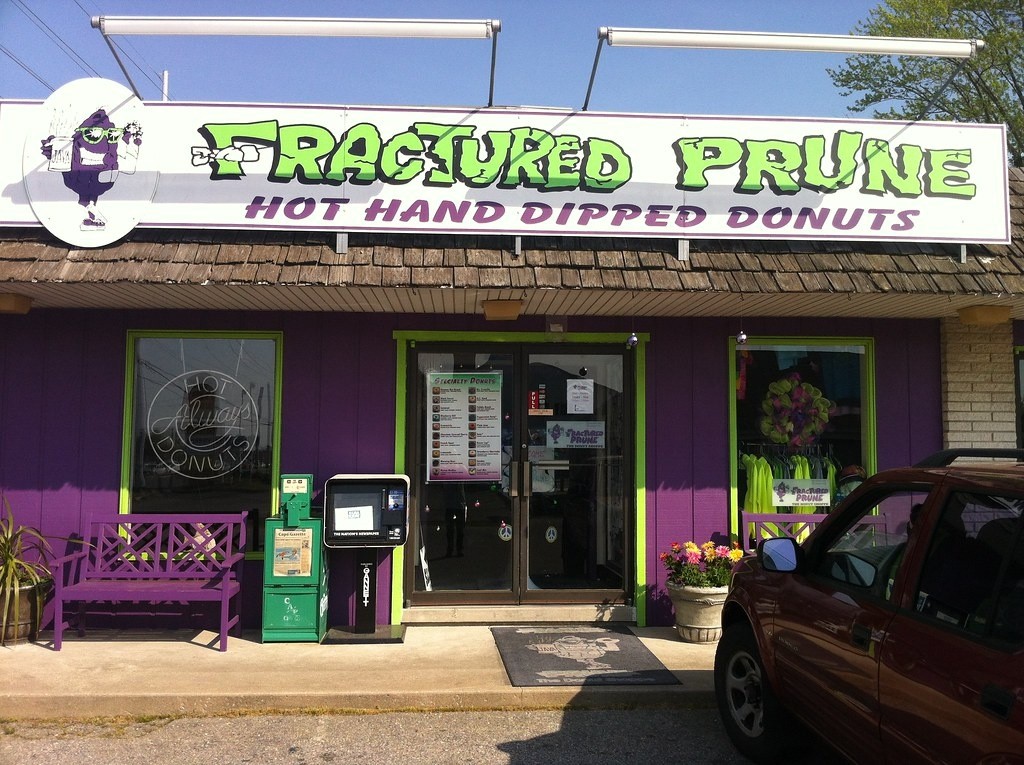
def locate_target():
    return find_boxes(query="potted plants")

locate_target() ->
[0,494,97,646]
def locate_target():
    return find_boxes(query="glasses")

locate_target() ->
[907,522,913,535]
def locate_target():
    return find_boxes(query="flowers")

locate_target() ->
[659,539,743,586]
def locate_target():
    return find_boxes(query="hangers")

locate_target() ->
[738,442,842,474]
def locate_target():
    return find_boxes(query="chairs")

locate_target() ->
[904,504,1024,629]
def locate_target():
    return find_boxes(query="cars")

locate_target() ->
[714,446,1024,765]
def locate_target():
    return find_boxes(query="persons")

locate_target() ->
[439,448,470,557]
[878,503,978,606]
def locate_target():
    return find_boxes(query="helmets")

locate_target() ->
[839,464,867,485]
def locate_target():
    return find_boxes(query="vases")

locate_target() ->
[666,580,730,644]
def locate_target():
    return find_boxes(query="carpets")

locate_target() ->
[491,625,684,686]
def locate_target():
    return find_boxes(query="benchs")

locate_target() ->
[50,511,249,651]
[742,511,890,557]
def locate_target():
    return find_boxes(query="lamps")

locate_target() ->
[90,15,500,108]
[583,26,984,121]
